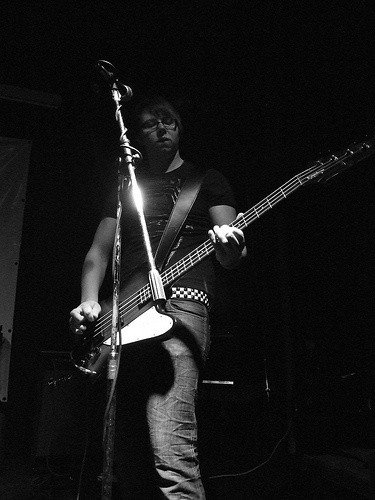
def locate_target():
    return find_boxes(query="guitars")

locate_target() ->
[69,138,372,379]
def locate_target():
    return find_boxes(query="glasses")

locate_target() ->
[136,117,177,134]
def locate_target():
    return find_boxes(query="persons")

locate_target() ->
[67,92,247,500]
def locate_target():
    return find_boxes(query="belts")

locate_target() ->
[166,287,209,310]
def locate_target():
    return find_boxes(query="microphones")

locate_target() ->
[115,77,133,102]
